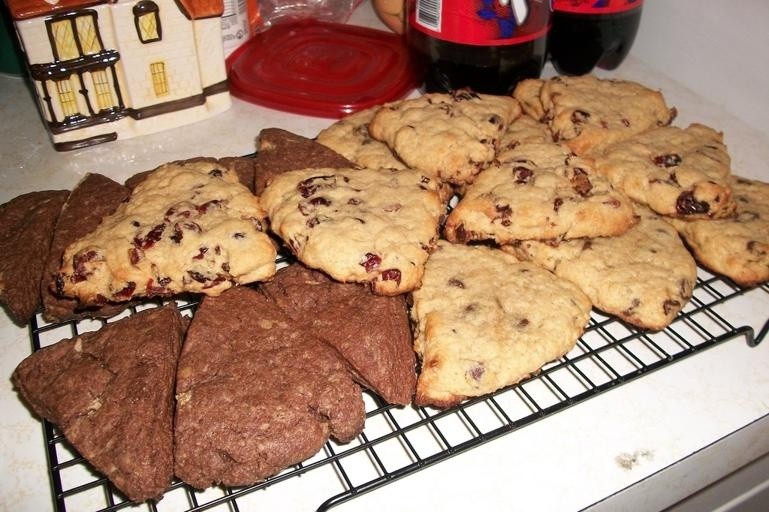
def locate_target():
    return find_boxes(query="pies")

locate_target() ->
[0,75,769,504]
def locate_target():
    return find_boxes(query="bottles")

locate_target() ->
[405,1,644,95]
